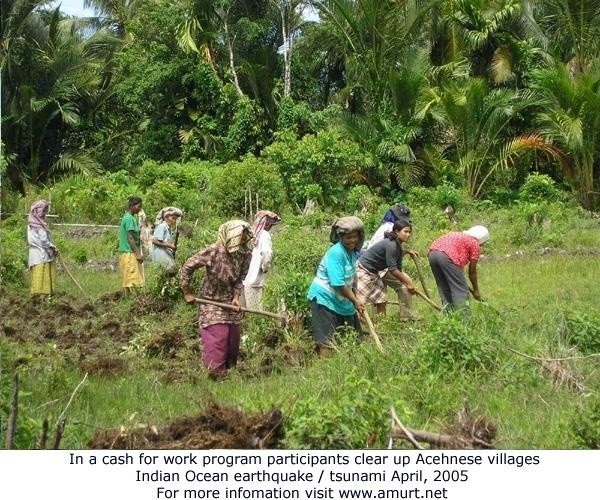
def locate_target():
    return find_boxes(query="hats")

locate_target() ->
[390,204,411,224]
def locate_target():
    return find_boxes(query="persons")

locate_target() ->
[306,216,365,359]
[427,224,489,318]
[354,219,415,320]
[27,199,58,295]
[118,197,145,296]
[179,220,255,381]
[239,210,282,310]
[367,203,422,322]
[152,207,183,274]
